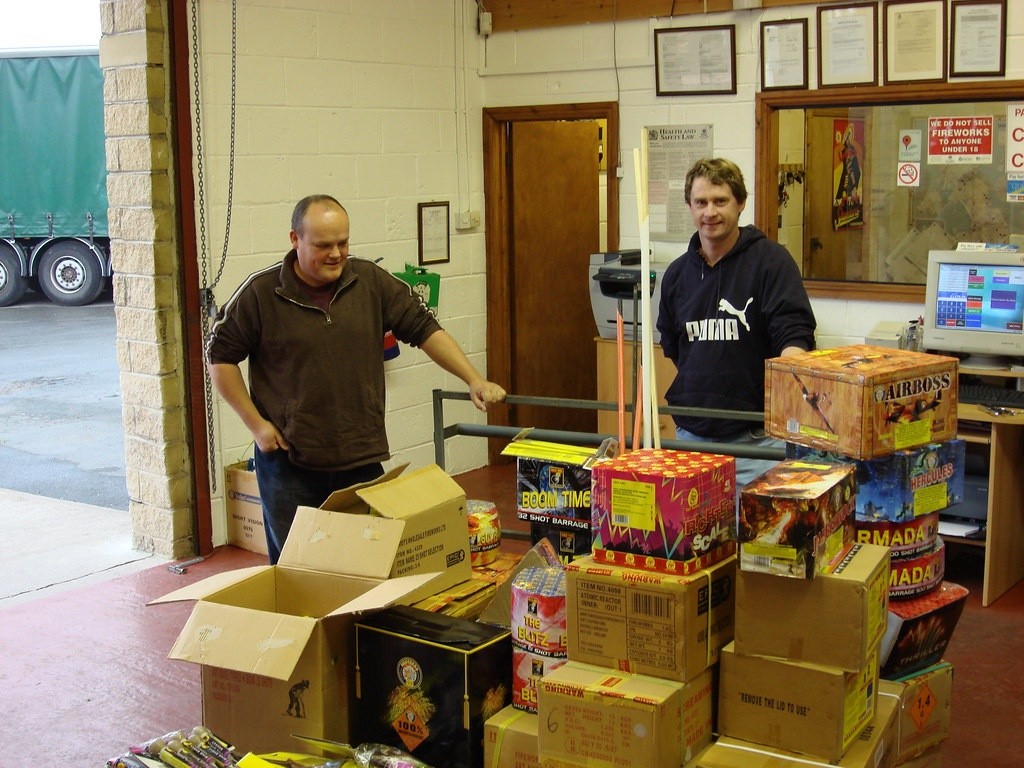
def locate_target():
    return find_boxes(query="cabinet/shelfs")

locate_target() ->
[593,335,679,440]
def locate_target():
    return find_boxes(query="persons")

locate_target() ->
[205,194,506,566]
[656,156,817,534]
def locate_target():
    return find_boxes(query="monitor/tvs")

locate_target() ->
[924,249,1024,370]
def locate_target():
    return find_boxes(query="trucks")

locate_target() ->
[0,46,115,306]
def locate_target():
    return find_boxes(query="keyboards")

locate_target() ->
[958,383,1024,409]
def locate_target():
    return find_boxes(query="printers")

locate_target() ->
[938,474,990,542]
[588,251,674,344]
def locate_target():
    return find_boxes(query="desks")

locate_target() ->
[937,368,1024,607]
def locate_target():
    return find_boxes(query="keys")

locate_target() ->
[779,170,805,208]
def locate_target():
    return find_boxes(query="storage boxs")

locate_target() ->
[142,341,970,768]
